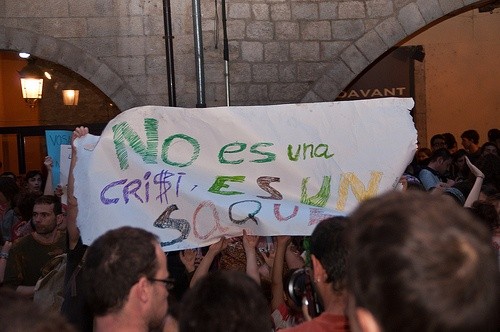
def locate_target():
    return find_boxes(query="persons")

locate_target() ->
[80,226,179,332]
[0,126,500,332]
[341,190,500,331]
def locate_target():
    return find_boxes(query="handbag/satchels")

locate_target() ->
[32,246,83,316]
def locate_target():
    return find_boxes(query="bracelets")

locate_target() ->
[0,252,9,260]
[477,175,484,179]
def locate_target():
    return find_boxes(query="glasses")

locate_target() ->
[148,272,177,290]
[432,143,444,147]
